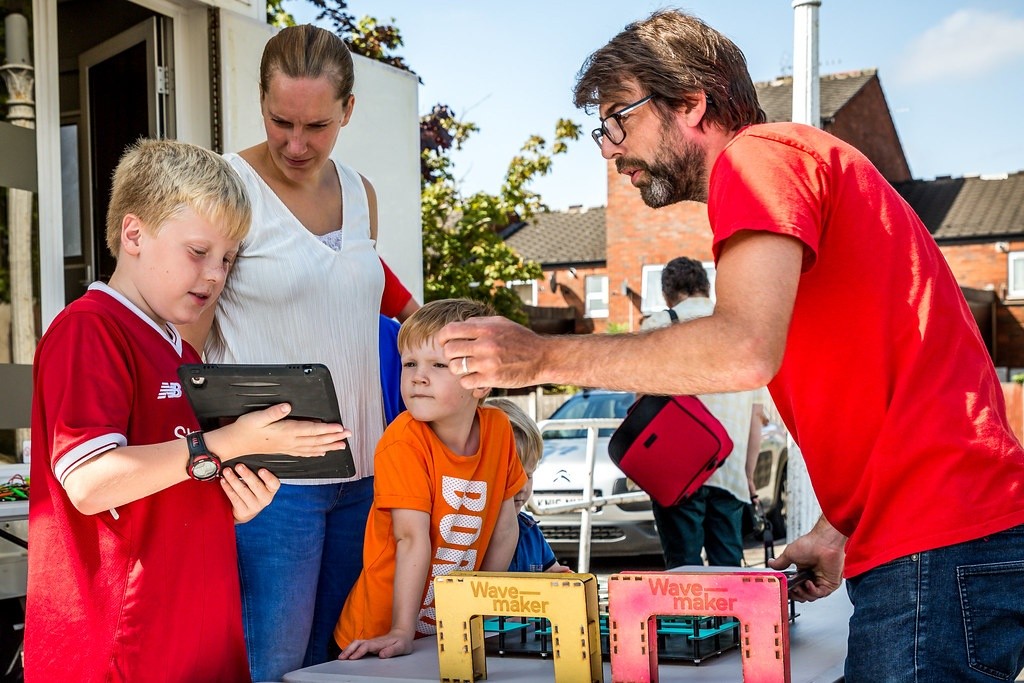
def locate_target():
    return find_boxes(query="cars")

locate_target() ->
[519,389,787,571]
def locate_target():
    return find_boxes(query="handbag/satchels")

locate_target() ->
[608,394,734,509]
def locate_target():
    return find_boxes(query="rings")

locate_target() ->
[462,356,470,374]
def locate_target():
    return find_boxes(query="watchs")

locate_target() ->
[185,431,221,483]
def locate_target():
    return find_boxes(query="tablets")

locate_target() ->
[176,363,356,480]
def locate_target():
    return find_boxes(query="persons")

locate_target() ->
[439,7,1022,682]
[21,140,351,683]
[640,257,763,572]
[170,26,574,683]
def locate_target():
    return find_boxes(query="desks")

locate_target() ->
[279,563,854,683]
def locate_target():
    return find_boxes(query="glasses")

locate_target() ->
[591,91,713,149]
